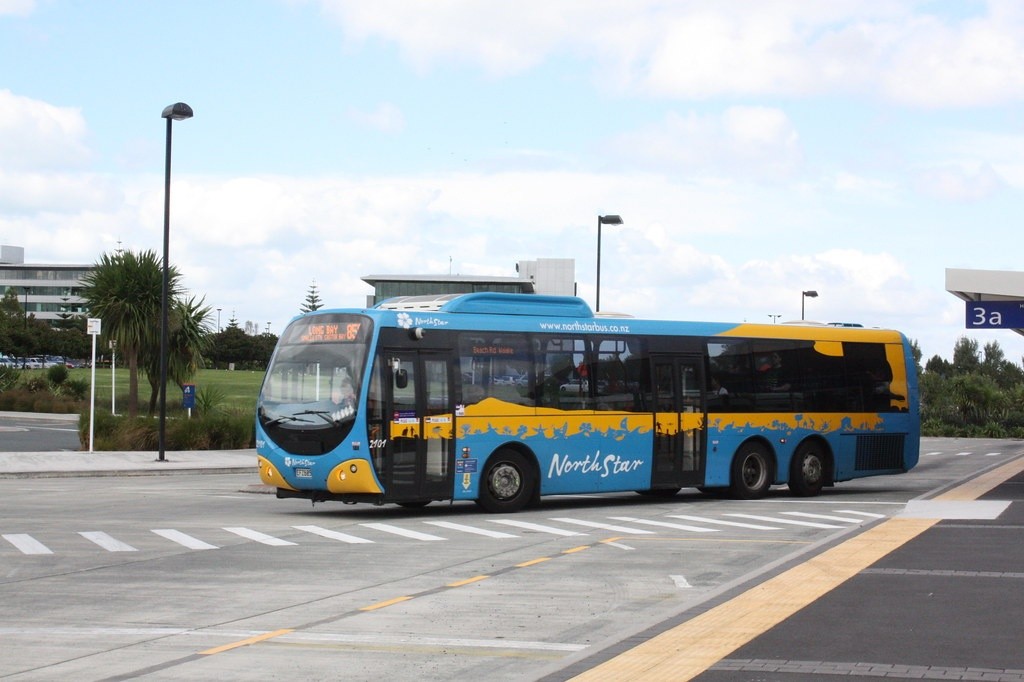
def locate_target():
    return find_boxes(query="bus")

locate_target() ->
[255,293,921,516]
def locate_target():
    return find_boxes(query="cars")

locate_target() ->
[488,374,528,392]
[0,356,77,371]
[463,372,478,383]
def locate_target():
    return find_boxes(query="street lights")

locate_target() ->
[802,291,818,321]
[596,214,624,313]
[23,286,30,368]
[158,102,193,461]
[217,308,222,333]
[768,314,781,324]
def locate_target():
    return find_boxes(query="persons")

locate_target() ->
[466,350,799,411]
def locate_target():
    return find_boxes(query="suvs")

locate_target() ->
[559,379,637,392]
